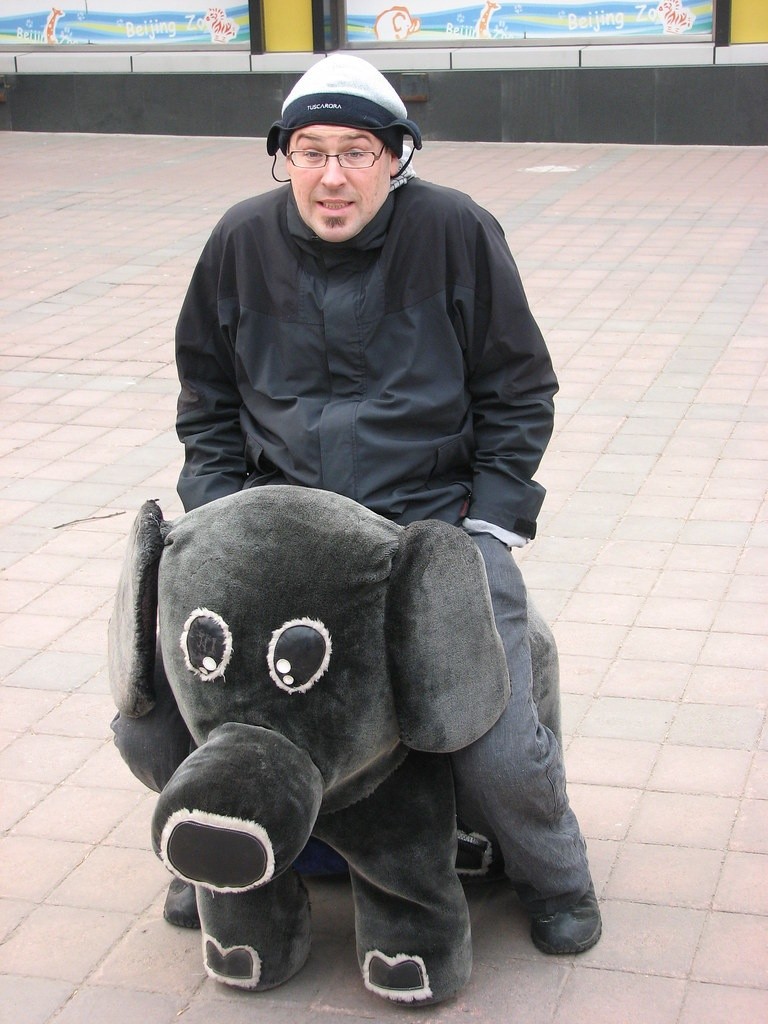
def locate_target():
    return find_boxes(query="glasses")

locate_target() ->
[285,143,386,168]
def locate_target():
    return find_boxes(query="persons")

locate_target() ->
[165,56,603,955]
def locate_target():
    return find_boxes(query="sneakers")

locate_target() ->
[164,874,204,929]
[531,887,601,955]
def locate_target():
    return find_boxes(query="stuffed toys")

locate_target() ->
[107,485,562,1007]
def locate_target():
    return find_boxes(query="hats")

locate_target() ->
[267,53,422,160]
